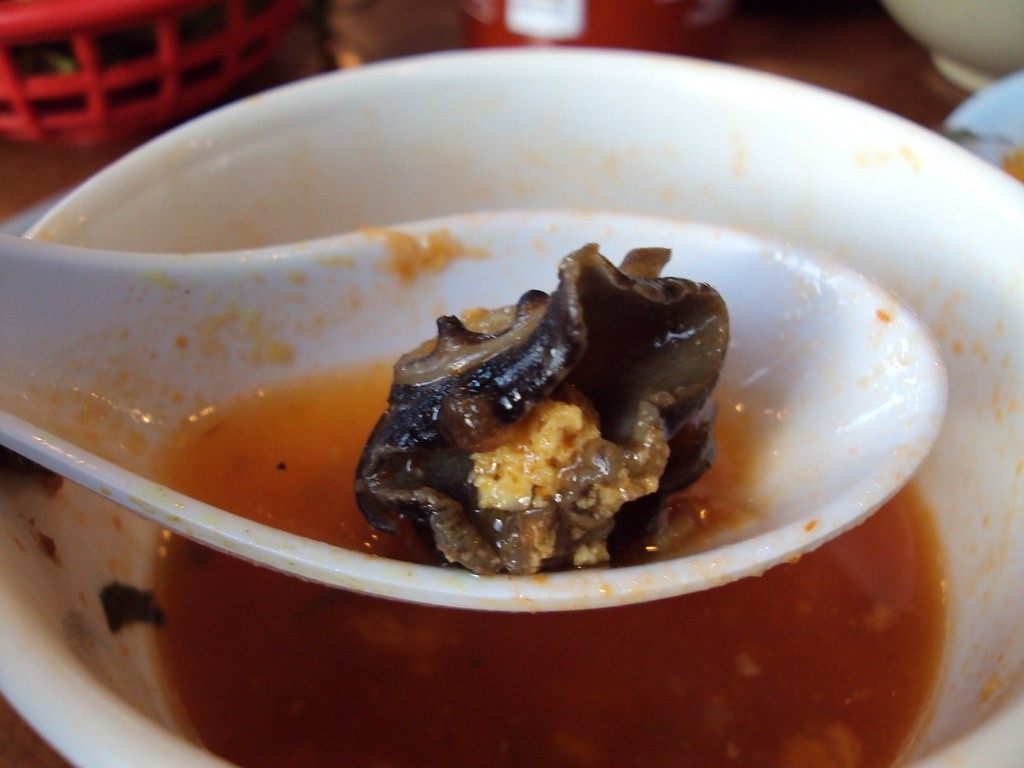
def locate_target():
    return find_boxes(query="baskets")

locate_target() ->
[0,0,327,149]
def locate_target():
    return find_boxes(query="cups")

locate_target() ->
[464,0,731,54]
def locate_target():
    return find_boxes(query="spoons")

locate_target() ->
[0,210,946,613]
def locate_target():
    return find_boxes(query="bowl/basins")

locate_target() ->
[0,48,1024,768]
[885,0,1024,89]
